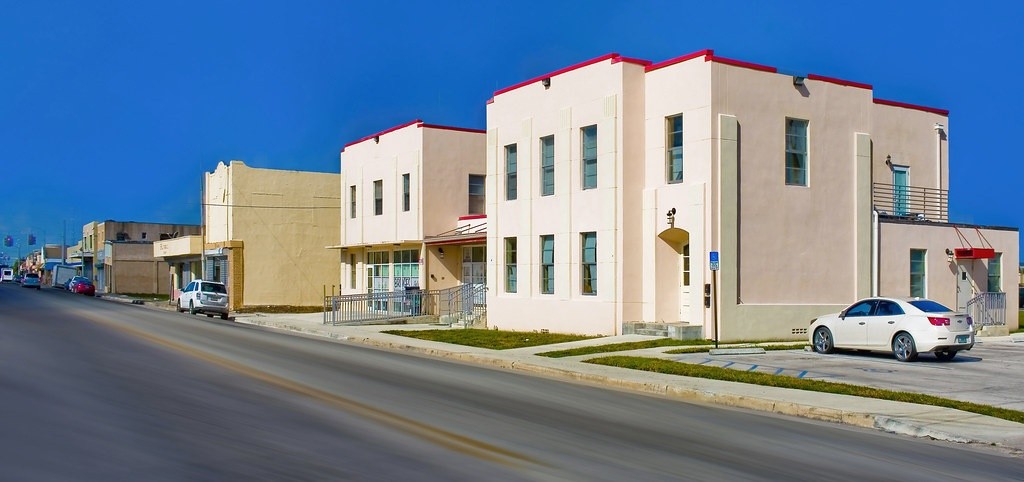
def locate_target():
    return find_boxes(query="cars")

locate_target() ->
[807,296,974,362]
[20,272,41,290]
[68,276,88,291]
[72,279,95,296]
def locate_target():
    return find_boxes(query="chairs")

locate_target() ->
[876,302,892,315]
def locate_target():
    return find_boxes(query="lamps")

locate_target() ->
[542,77,550,89]
[946,247,954,262]
[886,154,891,165]
[438,248,444,258]
[793,74,805,86]
[374,136,379,144]
[667,208,676,224]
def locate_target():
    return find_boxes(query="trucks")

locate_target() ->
[51,264,77,289]
[1,268,14,283]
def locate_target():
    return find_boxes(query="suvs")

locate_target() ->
[177,279,230,320]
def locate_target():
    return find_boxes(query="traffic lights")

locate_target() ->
[28,233,36,246]
[4,236,13,248]
